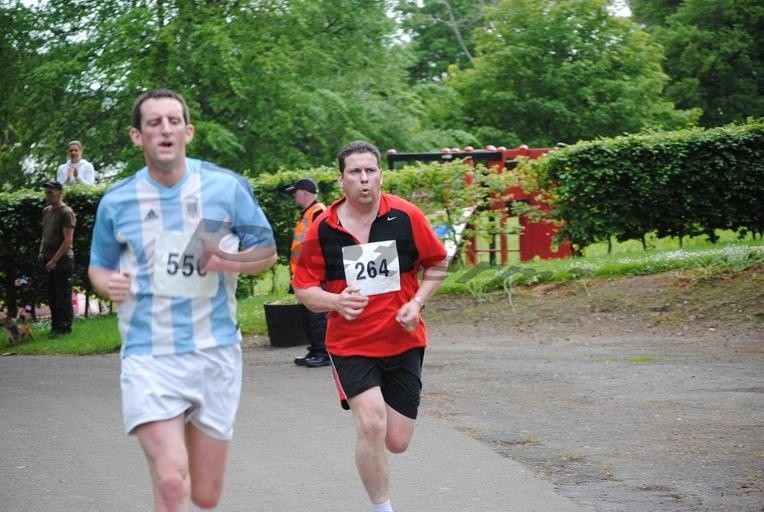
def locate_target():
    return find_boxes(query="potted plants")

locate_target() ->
[259,289,309,349]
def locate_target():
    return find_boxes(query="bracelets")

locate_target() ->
[39,251,43,254]
[51,258,56,264]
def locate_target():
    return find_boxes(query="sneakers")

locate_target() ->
[49,325,72,339]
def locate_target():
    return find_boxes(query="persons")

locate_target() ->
[286,179,331,368]
[85,88,280,511]
[35,180,76,339]
[294,139,447,511]
[56,141,95,188]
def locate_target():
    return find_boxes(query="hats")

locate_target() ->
[42,180,63,191]
[285,178,318,194]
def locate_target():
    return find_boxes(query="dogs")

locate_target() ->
[6,317,34,346]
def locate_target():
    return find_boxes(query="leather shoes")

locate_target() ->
[294,346,315,366]
[305,352,331,368]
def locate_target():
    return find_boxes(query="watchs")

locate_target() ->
[411,295,426,313]
